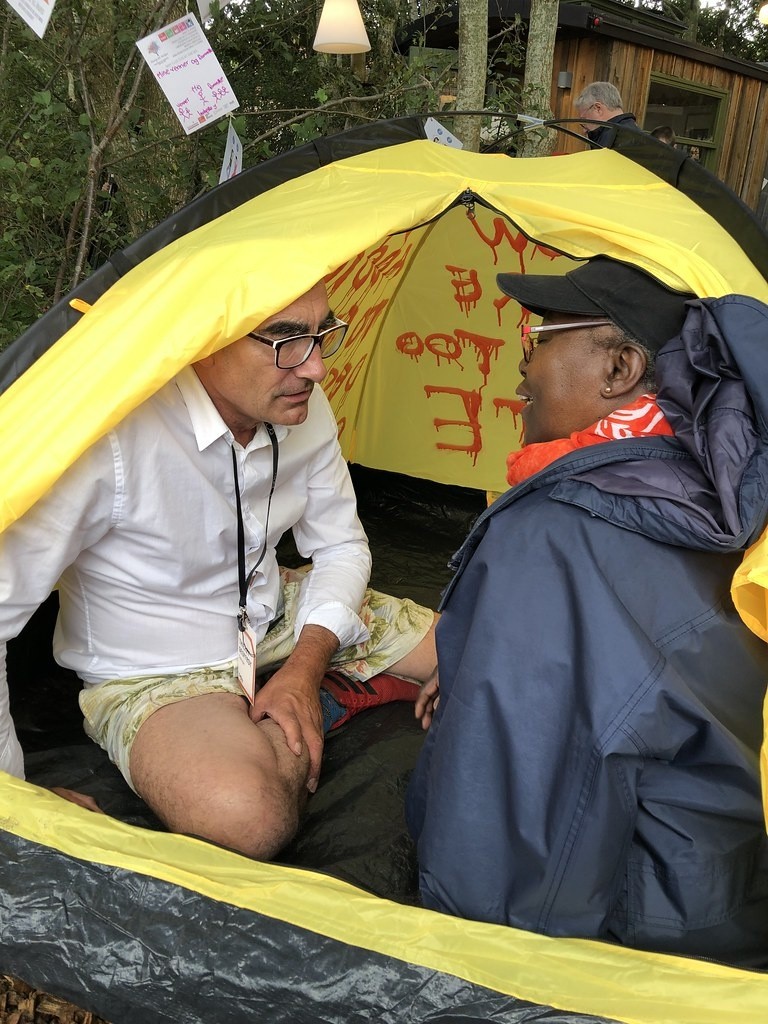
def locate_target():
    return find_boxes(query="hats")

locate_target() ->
[495,257,698,356]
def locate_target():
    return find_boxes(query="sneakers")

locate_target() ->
[320,670,424,729]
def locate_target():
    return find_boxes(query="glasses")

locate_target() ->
[520,322,613,364]
[578,105,596,129]
[246,316,349,371]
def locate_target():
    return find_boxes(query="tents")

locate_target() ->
[0,110,768,1024]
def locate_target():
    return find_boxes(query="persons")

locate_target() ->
[0,276,451,862]
[570,82,646,152]
[403,254,767,973]
[649,124,679,150]
